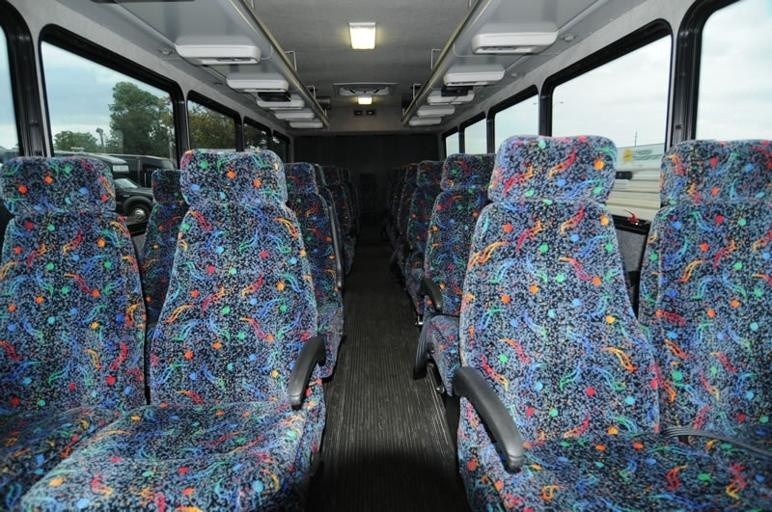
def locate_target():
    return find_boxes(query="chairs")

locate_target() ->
[388,135,770,511]
[1,149,356,512]
